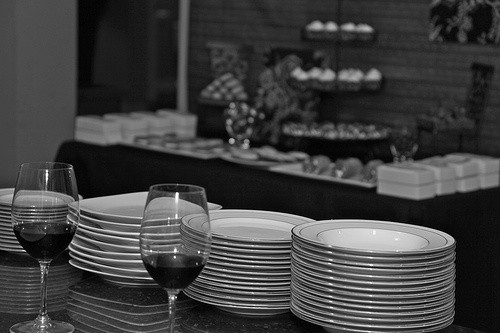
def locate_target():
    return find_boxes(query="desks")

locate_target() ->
[0,253,478,333]
[51,137,500,333]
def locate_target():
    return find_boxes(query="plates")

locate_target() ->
[137,141,220,161]
[0,187,458,333]
[266,161,377,188]
[217,153,281,168]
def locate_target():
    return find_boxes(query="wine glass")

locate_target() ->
[139,183,211,333]
[11,162,80,333]
